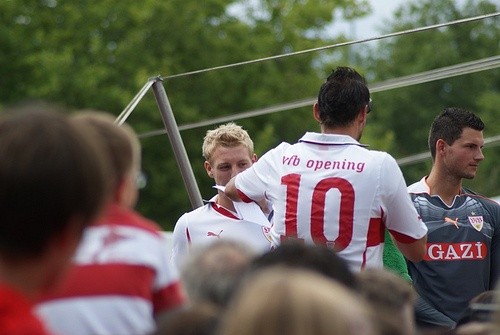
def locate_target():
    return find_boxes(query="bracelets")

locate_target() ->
[266,209,274,222]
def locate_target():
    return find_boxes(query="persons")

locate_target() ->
[34,110,189,334]
[401,106,500,335]
[1,102,107,334]
[172,122,274,273]
[223,67,428,273]
[141,240,499,335]
